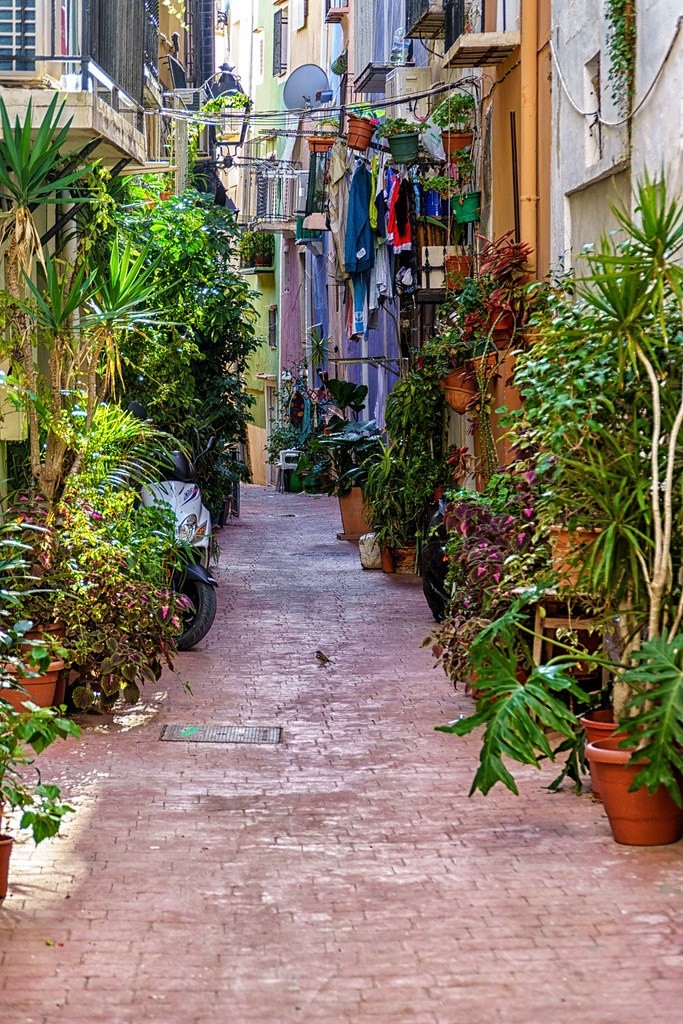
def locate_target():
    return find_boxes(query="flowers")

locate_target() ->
[345,109,386,124]
[376,116,432,139]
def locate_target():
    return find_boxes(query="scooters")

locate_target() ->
[127,401,220,651]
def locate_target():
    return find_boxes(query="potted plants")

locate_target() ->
[462,336,499,378]
[434,93,475,163]
[430,595,683,846]
[409,329,476,393]
[293,421,384,538]
[417,215,472,290]
[532,151,683,799]
[475,229,538,329]
[423,444,457,500]
[345,444,404,575]
[0,477,221,899]
[365,487,419,573]
[307,118,341,153]
[421,145,482,224]
[237,231,273,267]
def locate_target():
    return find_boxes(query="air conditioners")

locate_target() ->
[173,88,211,157]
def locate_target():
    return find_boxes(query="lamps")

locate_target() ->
[159,63,253,169]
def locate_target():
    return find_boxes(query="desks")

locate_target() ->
[509,589,614,728]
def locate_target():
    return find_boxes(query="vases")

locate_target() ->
[550,526,606,582]
[388,132,419,164]
[347,118,378,150]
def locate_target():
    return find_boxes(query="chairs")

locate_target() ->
[279,450,304,493]
